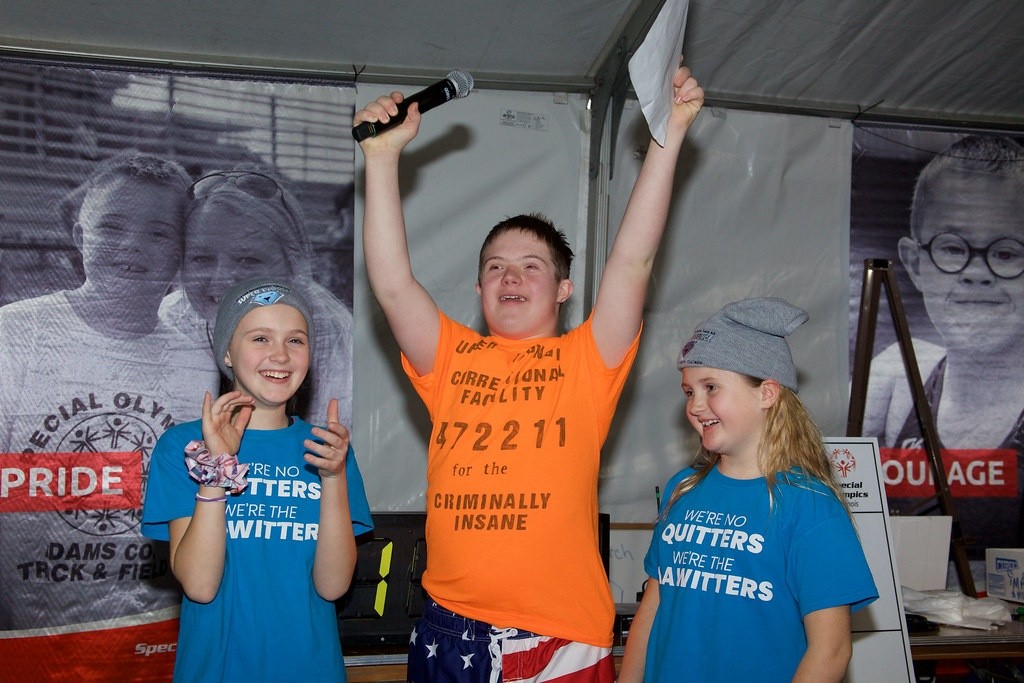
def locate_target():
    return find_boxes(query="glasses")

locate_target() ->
[918,231,1023,279]
[189,170,288,209]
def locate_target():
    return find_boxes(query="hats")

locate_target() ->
[676,298,810,391]
[213,276,315,383]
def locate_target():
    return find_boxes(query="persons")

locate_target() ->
[140,272,378,683]
[154,156,361,580]
[0,152,224,630]
[350,48,705,683]
[853,132,1024,511]
[618,295,881,683]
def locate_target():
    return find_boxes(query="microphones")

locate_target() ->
[353,69,474,143]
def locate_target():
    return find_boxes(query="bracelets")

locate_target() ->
[194,490,229,503]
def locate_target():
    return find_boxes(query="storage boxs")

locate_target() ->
[985,547,1024,604]
